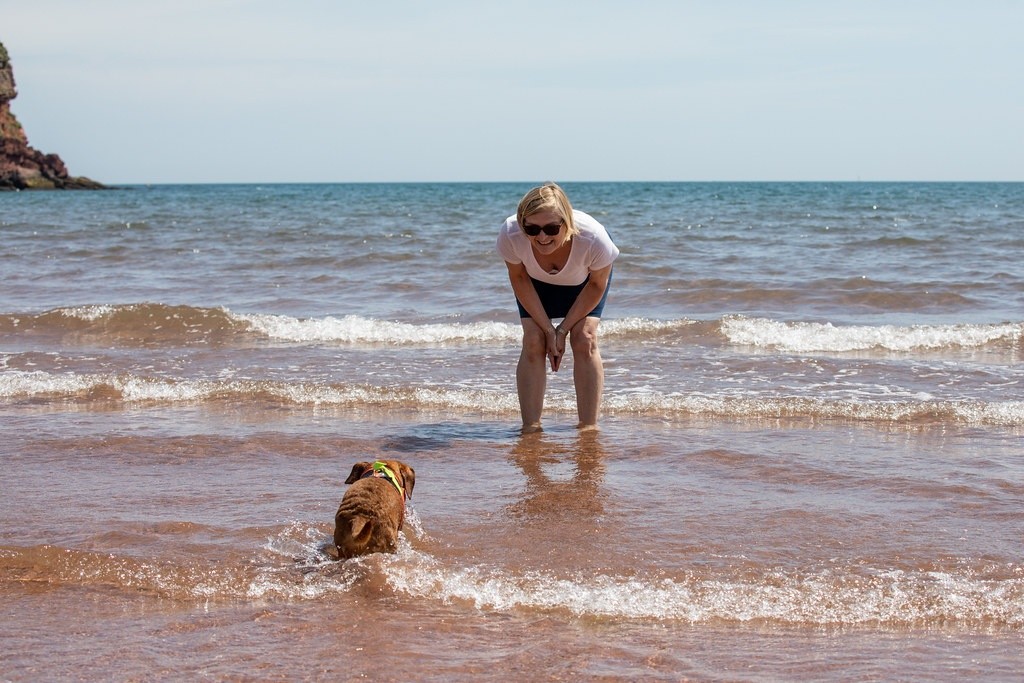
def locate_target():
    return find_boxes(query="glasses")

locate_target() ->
[523,221,564,236]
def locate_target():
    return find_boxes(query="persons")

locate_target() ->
[495,183,620,426]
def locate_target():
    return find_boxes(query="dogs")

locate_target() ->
[333,459,417,558]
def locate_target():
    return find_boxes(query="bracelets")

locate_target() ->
[557,325,568,336]
